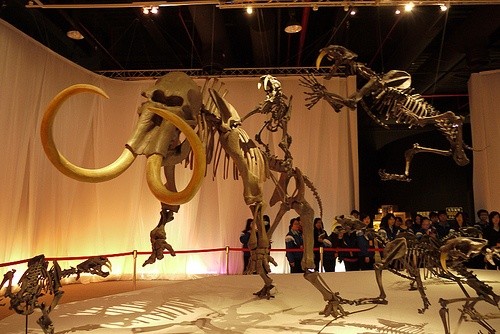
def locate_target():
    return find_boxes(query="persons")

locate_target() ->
[239,219,258,274]
[285,210,500,275]
[262,215,273,273]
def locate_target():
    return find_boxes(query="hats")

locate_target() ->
[320,232,325,239]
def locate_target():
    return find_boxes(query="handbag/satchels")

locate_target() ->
[334,257,346,272]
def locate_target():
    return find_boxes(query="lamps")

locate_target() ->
[284,17,303,34]
[67,27,84,40]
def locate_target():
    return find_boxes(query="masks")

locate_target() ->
[264,221,269,225]
[480,216,488,220]
